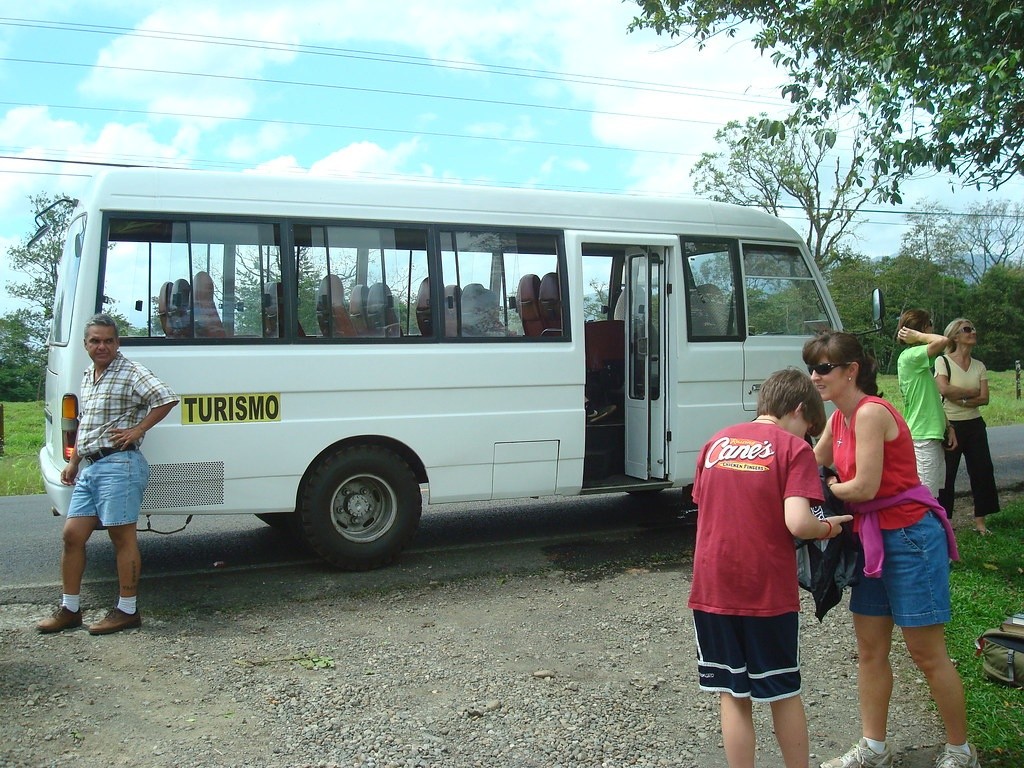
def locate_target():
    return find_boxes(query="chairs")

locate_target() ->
[158,271,736,390]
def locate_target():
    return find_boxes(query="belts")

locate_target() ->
[84,443,134,465]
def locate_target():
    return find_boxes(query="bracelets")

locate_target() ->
[947,425,954,433]
[816,519,832,541]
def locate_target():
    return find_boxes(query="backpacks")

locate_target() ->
[978,624,1024,691]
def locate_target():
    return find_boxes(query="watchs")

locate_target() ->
[961,398,967,407]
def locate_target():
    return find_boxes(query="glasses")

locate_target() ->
[807,362,850,375]
[956,327,975,334]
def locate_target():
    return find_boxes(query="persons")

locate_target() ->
[36,314,180,634]
[803,333,980,768]
[688,368,853,768]
[933,319,1000,535]
[897,308,958,498]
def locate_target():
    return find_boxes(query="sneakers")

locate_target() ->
[935,742,981,768]
[89,606,141,635]
[36,605,82,632]
[819,738,894,768]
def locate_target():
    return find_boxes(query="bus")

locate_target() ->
[26,166,887,573]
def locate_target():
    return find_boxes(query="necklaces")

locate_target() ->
[837,413,850,446]
[756,418,776,424]
